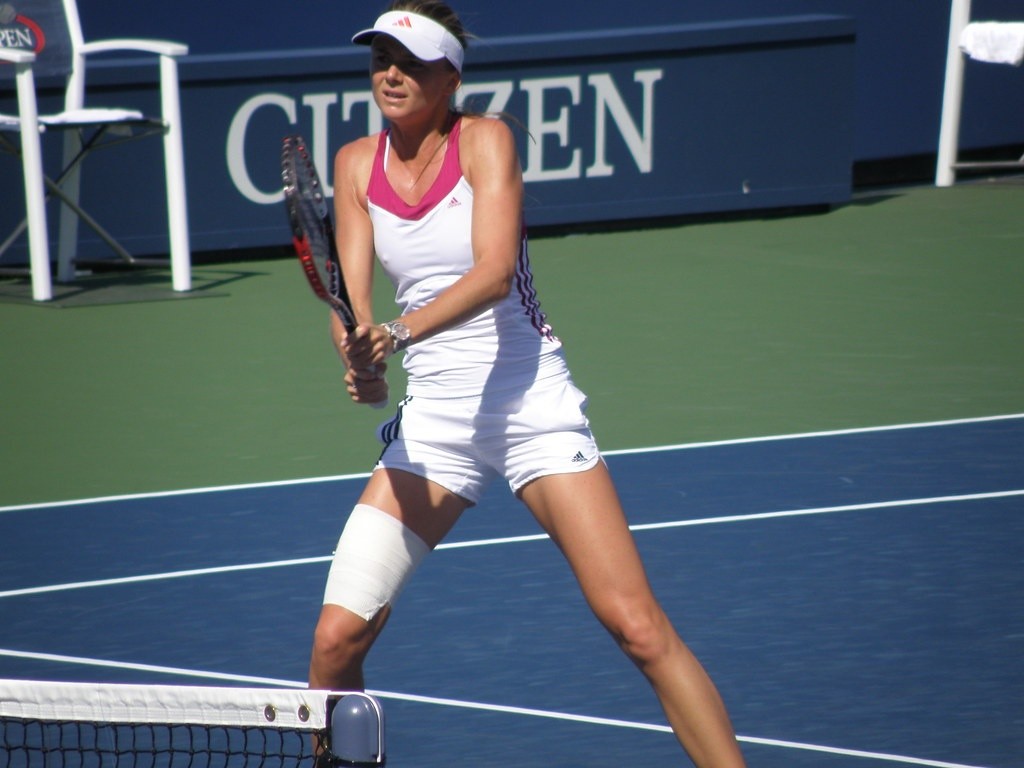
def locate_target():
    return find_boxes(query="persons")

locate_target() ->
[306,0,749,767]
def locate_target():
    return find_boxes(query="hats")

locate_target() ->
[352,10,465,74]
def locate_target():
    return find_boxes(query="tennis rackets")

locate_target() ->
[280,135,388,409]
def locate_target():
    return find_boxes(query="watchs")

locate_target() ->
[381,319,412,352]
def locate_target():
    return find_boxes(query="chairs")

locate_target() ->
[0,0,190,300]
[935,0,1024,187]
